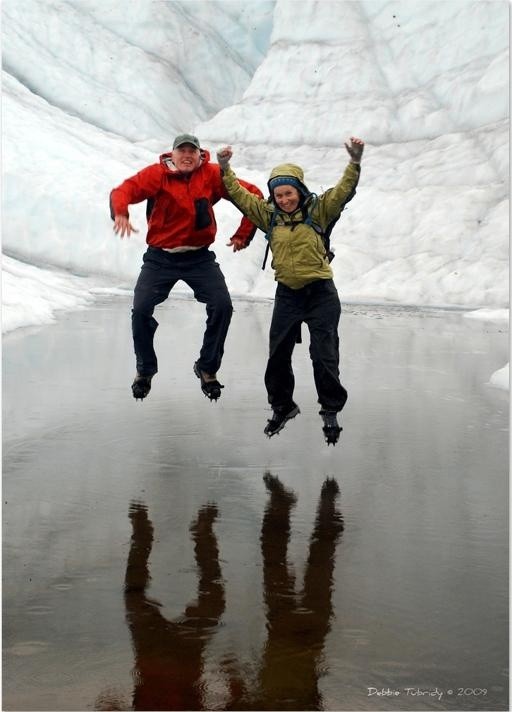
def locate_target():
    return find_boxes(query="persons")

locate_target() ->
[236,470,345,711]
[107,130,263,401]
[216,136,365,446]
[97,497,247,711]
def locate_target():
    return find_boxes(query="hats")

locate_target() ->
[173,134,200,151]
[269,165,307,198]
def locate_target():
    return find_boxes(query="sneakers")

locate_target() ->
[318,408,342,446]
[193,363,224,402]
[131,371,157,398]
[263,401,300,438]
